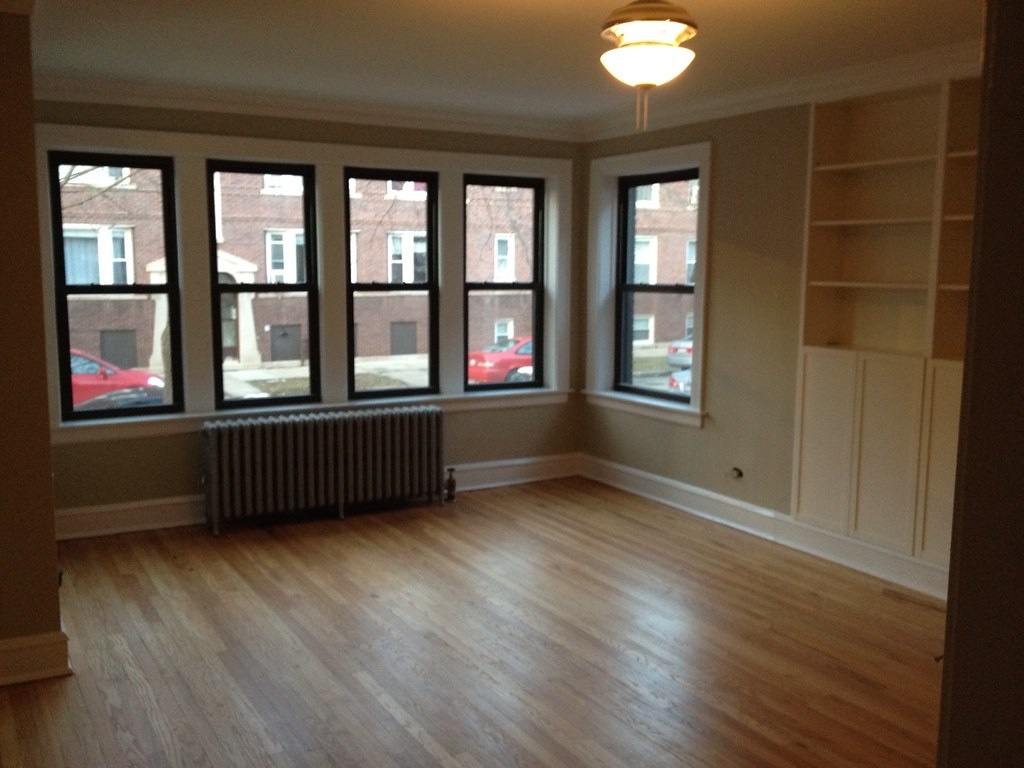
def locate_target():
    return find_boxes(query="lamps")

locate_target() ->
[598,0,698,132]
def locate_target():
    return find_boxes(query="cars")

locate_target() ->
[71,385,235,410]
[69,348,166,409]
[669,370,690,396]
[467,337,533,383]
[667,333,694,370]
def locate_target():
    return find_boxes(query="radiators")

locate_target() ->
[199,403,456,536]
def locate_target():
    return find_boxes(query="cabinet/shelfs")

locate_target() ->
[789,71,982,570]
